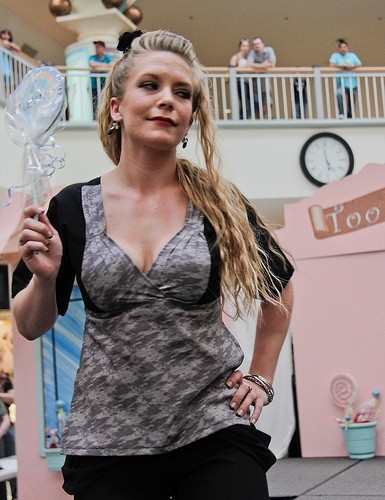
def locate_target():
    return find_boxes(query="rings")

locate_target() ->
[245,383,252,391]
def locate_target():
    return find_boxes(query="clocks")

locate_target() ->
[300,132,354,186]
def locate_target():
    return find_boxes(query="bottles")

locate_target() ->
[363,390,381,422]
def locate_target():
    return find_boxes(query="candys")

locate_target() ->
[5,65,64,253]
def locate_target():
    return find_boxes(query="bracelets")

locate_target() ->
[243,372,274,405]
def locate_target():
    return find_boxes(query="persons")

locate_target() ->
[246,36,277,120]
[0,371,18,500]
[8,31,296,500]
[0,29,21,98]
[89,40,114,120]
[328,41,362,119]
[292,77,308,119]
[229,38,251,120]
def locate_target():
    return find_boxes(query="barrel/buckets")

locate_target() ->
[339,420,377,460]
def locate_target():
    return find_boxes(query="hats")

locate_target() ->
[93,40,105,47]
